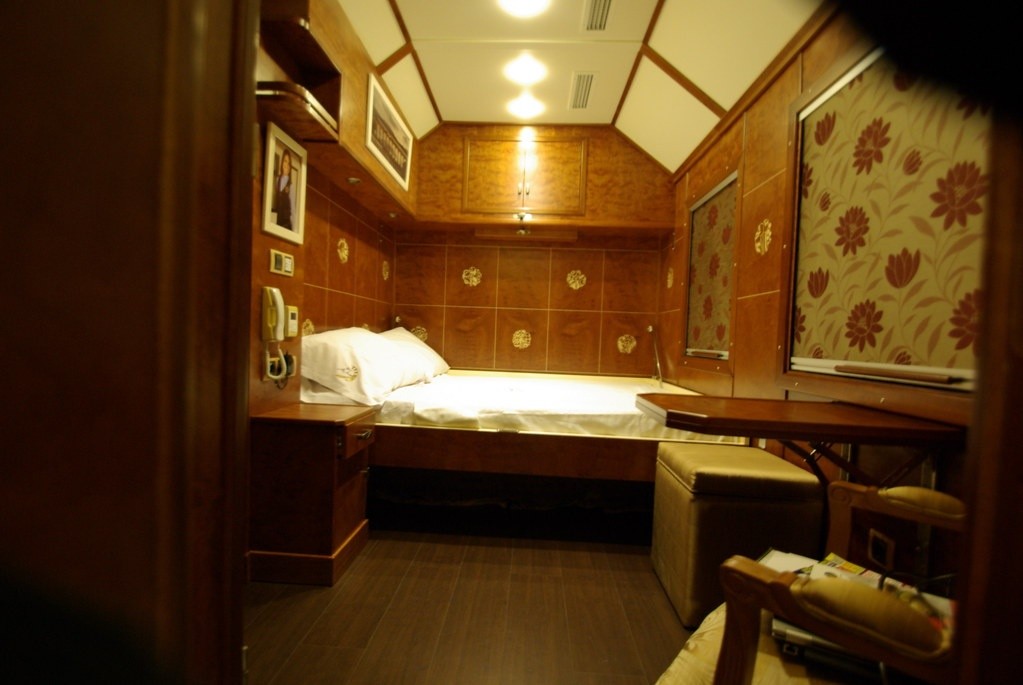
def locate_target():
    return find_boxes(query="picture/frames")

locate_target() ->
[364,72,413,192]
[262,121,308,246]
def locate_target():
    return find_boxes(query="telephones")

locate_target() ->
[261,286,285,343]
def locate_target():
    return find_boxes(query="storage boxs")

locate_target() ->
[653,441,823,629]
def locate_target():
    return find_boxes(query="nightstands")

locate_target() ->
[250,403,376,586]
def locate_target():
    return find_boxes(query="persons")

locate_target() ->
[273,149,294,231]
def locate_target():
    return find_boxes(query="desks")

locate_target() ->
[636,393,969,591]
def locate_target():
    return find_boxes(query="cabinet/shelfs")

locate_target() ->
[415,120,673,237]
[255,1,419,228]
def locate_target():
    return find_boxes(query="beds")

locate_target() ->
[301,370,750,482]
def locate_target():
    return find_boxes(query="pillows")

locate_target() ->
[301,327,450,408]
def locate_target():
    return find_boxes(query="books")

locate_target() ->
[754,539,961,681]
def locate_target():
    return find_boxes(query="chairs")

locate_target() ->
[658,124,1023,685]
